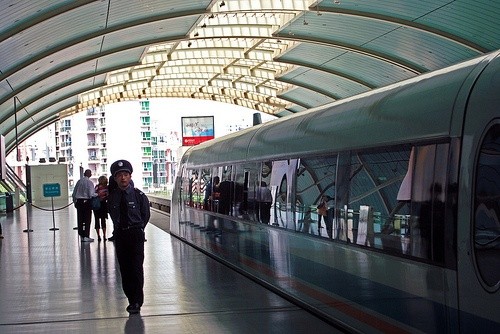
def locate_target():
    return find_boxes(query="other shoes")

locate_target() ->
[98,238,101,241]
[126,304,140,314]
[81,237,94,242]
[108,238,113,241]
[104,238,107,240]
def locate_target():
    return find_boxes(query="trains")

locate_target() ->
[168,48,500,334]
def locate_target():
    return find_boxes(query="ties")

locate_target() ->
[119,192,128,228]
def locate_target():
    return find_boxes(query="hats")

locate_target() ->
[110,160,133,177]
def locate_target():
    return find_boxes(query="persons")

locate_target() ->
[417,182,444,243]
[203,176,219,212]
[106,160,150,315]
[317,196,335,238]
[72,169,98,242]
[260,182,272,225]
[94,175,134,241]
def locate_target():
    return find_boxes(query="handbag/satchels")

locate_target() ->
[92,197,100,211]
